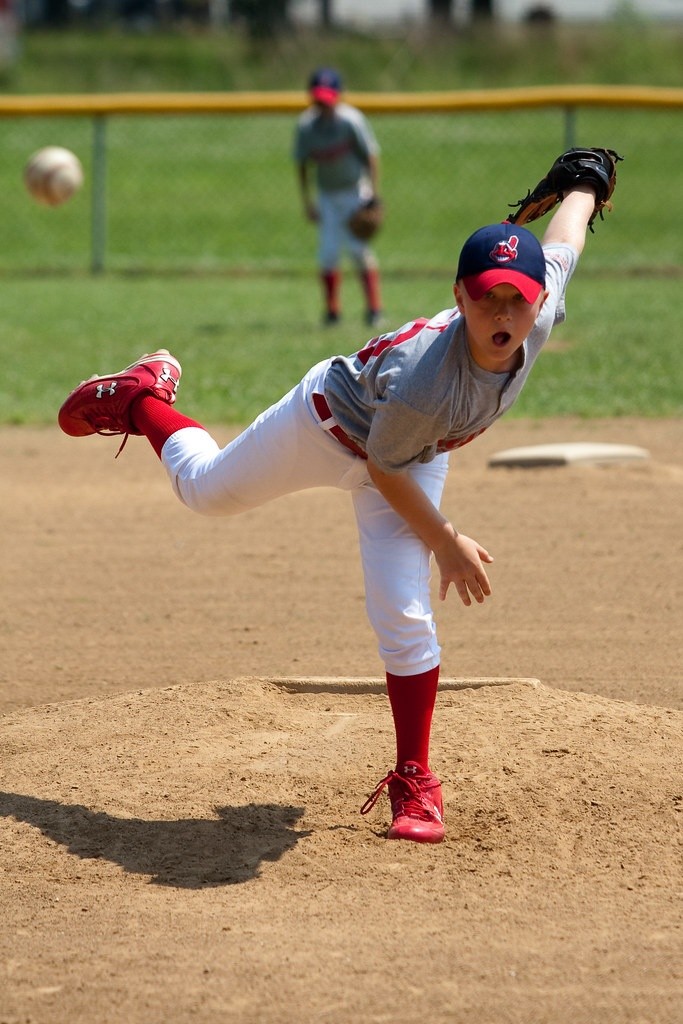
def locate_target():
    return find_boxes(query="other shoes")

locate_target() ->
[325,313,339,324]
[367,311,380,323]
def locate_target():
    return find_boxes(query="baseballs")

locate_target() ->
[23,147,84,204]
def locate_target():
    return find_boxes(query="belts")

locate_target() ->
[311,393,369,460]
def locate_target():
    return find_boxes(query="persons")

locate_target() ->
[295,74,381,327]
[58,148,598,842]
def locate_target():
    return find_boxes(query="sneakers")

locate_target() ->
[361,760,444,845]
[59,349,182,458]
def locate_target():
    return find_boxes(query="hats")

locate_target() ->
[305,69,340,106]
[456,223,546,303]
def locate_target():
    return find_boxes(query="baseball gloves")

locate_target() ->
[351,198,384,241]
[511,143,620,231]
